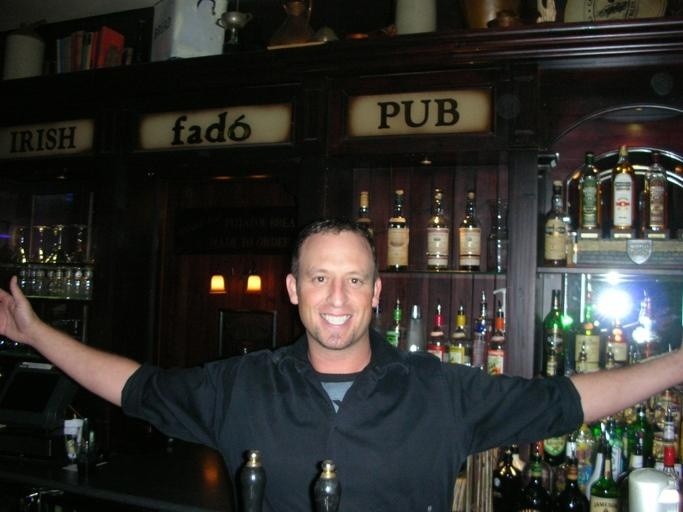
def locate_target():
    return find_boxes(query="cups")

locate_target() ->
[63,418,83,463]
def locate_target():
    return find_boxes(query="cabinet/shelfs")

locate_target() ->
[0,18,683,512]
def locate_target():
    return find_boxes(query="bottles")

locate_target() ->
[7,225,93,293]
[357,148,683,512]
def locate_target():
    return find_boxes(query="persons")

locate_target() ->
[0,217,683,510]
